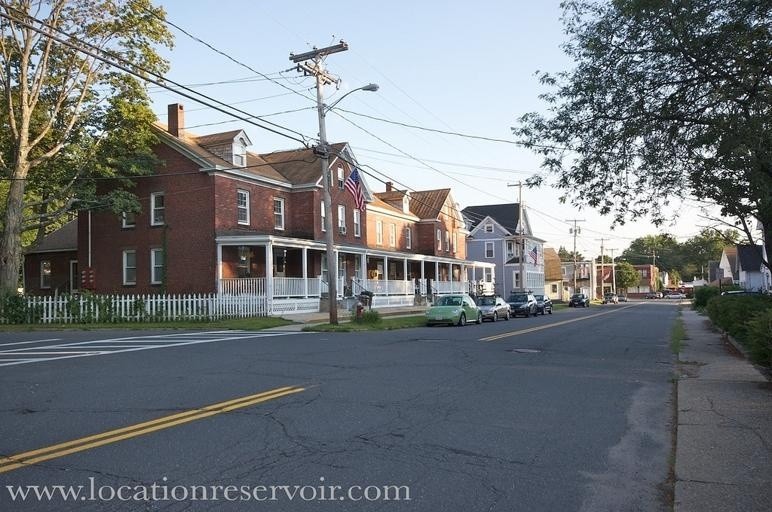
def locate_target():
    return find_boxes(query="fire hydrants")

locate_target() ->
[357,302,364,317]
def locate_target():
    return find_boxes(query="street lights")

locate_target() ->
[319,85,380,324]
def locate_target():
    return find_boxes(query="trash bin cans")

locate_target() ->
[358,291,375,310]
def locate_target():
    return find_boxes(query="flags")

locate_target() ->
[529,247,538,266]
[344,168,366,212]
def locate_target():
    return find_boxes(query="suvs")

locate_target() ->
[505,290,538,318]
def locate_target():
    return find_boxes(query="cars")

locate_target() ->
[645,290,686,298]
[424,293,482,326]
[602,292,627,304]
[475,294,511,321]
[569,293,589,307]
[534,295,553,315]
